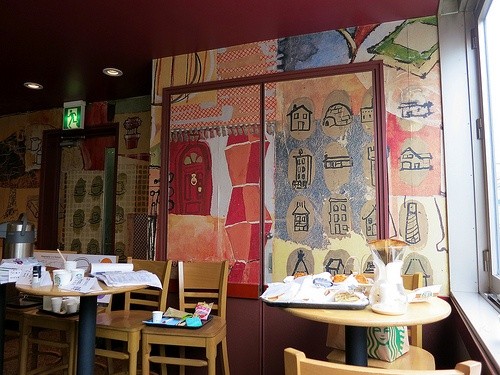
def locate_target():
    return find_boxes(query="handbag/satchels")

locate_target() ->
[326,254,410,362]
[369,245,409,315]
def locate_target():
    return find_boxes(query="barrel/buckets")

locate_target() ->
[5,213,35,258]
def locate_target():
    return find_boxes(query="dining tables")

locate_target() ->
[15,272,150,375]
[277,290,452,367]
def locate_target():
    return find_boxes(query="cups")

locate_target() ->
[50,296,79,313]
[72,269,84,279]
[152,311,164,323]
[369,246,407,315]
[53,269,71,286]
[63,261,77,270]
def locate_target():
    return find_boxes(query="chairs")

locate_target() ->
[0,250,482,375]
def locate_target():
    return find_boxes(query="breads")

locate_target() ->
[292,272,368,302]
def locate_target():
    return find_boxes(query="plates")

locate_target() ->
[283,275,295,283]
[331,276,375,287]
